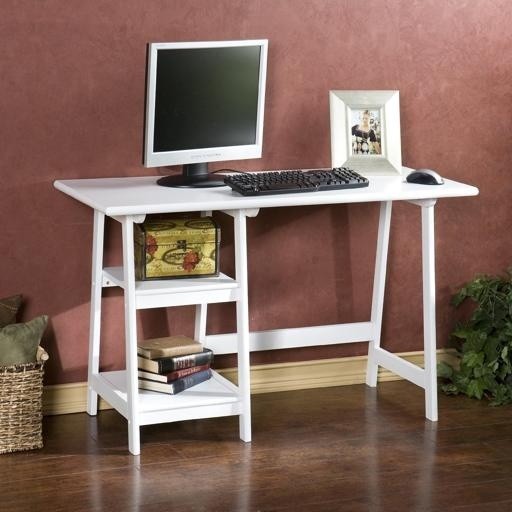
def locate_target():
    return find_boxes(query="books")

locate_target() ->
[132,334,213,395]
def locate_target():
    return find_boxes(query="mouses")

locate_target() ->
[406,168,445,185]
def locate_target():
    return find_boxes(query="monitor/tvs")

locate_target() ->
[142,39,270,188]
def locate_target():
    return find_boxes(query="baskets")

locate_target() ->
[0,346,50,453]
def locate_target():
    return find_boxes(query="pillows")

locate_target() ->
[0,293,49,373]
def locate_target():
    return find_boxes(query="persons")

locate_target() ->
[351,109,381,155]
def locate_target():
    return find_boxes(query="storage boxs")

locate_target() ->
[135,216,223,283]
[53,164,480,457]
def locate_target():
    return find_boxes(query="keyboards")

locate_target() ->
[223,166,369,197]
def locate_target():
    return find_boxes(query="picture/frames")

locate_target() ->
[329,89,403,176]
